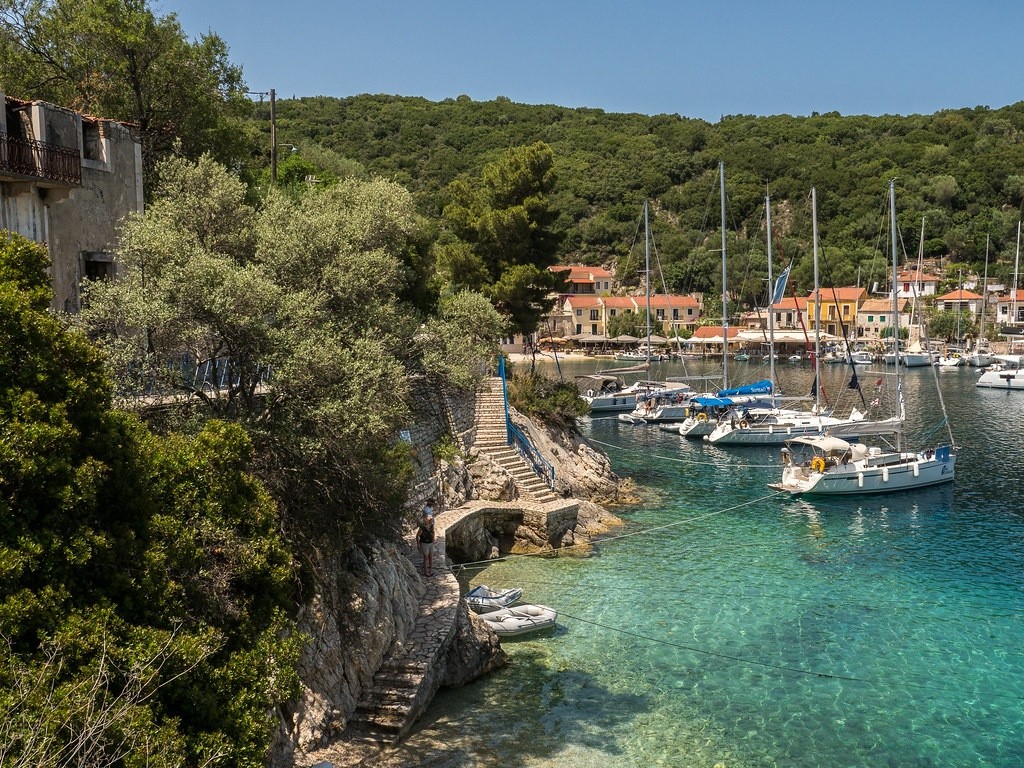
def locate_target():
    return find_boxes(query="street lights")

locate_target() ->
[269,142,297,183]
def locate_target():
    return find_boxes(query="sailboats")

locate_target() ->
[758,177,964,493]
[705,183,912,443]
[566,161,1024,439]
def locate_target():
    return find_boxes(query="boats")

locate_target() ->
[463,584,525,612]
[477,603,559,638]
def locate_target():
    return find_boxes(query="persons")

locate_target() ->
[417,508,434,576]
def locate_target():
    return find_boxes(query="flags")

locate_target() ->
[769,262,792,305]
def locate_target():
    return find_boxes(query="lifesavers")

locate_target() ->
[685,408,689,417]
[697,413,707,422]
[812,458,825,474]
[953,353,958,357]
[587,389,593,397]
[739,420,747,430]
